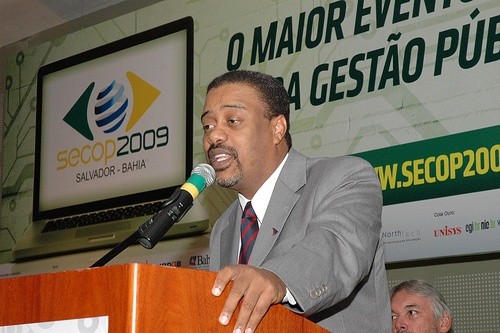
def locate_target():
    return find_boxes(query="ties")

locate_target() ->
[240,201,259,265]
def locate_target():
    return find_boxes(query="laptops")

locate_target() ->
[12,16,209,261]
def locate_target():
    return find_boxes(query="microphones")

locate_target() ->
[139,163,216,249]
